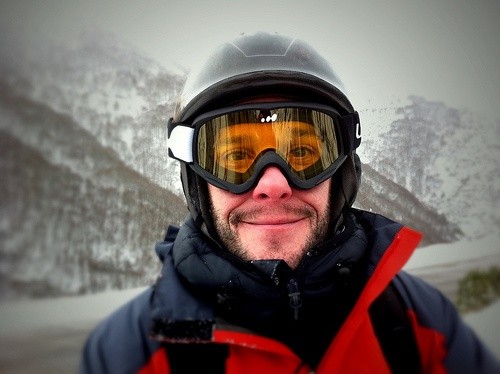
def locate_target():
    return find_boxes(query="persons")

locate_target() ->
[80,30,500,374]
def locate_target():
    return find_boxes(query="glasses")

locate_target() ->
[168,103,359,194]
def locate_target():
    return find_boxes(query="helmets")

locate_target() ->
[171,31,359,231]
